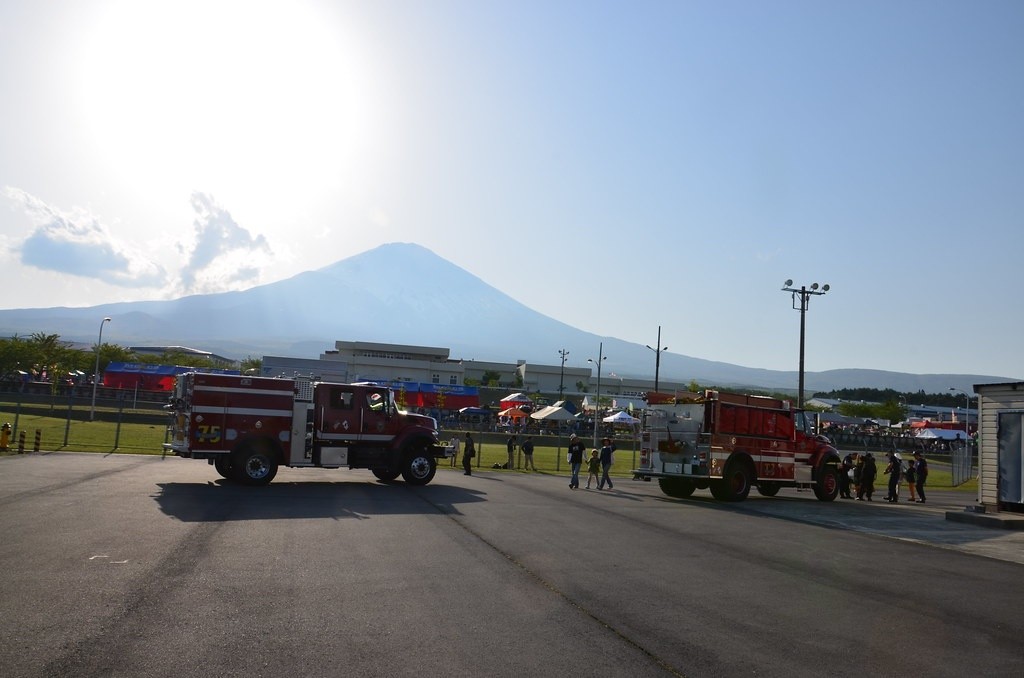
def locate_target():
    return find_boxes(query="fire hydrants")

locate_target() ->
[0,422,12,451]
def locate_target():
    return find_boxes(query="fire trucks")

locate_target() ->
[630,388,845,503]
[161,370,458,489]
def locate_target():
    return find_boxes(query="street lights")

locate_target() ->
[948,387,969,473]
[780,278,830,431]
[645,325,669,392]
[556,348,571,436]
[90,317,112,421]
[587,342,607,450]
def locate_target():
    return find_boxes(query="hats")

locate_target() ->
[911,451,922,456]
[885,450,894,456]
[570,434,579,441]
[602,437,612,444]
[591,449,599,455]
[895,453,903,460]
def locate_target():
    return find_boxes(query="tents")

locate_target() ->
[104,362,241,391]
[911,420,973,440]
[498,408,528,423]
[500,393,532,410]
[530,406,578,421]
[459,407,491,416]
[358,379,479,410]
[603,411,641,424]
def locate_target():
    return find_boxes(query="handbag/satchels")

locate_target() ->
[471,450,476,458]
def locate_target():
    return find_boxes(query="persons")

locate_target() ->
[462,432,474,475]
[491,416,520,434]
[567,434,587,489]
[450,434,460,467]
[882,451,907,502]
[586,449,600,489]
[912,450,928,503]
[841,452,877,502]
[904,460,916,501]
[508,435,516,469]
[598,437,614,490]
[521,436,534,471]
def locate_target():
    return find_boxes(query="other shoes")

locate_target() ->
[916,500,926,503]
[595,486,599,489]
[868,497,872,501]
[569,483,573,489]
[463,472,470,476]
[585,486,590,489]
[598,487,602,490]
[573,486,578,488]
[607,486,613,490]
[840,496,854,499]
[855,497,863,501]
[882,496,892,500]
